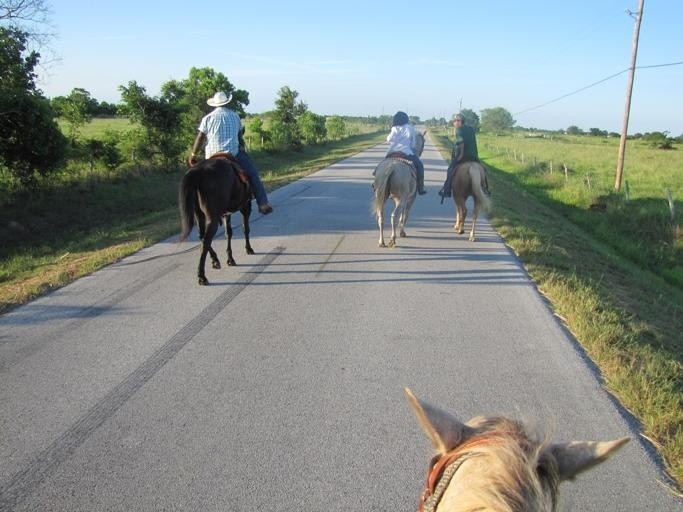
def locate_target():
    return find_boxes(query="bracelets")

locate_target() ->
[191,153,197,157]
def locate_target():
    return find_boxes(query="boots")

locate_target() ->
[417,176,426,195]
[481,179,491,194]
[439,183,451,198]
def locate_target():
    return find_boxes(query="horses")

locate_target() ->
[178,125,254,285]
[403,385,633,512]
[368,131,426,249]
[447,159,493,242]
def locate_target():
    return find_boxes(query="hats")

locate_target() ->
[452,114,465,122]
[207,91,233,107]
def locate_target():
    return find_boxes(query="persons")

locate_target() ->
[371,111,426,194]
[438,113,491,197]
[189,92,273,215]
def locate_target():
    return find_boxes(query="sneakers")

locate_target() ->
[259,202,273,214]
